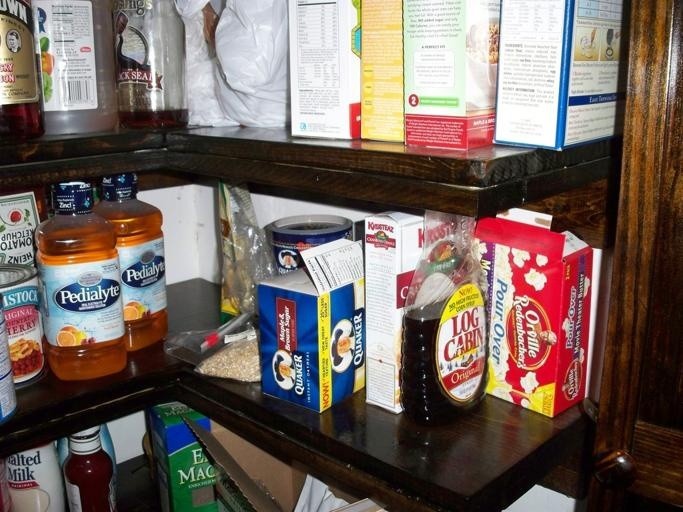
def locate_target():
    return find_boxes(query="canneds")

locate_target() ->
[0,262,45,425]
[270,214,353,277]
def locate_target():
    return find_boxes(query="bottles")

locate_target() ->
[0,423,117,512]
[33,174,167,382]
[402,245,486,425]
[1,0,186,141]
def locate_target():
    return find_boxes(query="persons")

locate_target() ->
[331,326,354,367]
[278,251,297,270]
[534,320,557,346]
[6,31,21,54]
[273,353,296,386]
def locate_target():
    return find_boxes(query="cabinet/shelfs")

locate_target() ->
[1,119,625,512]
